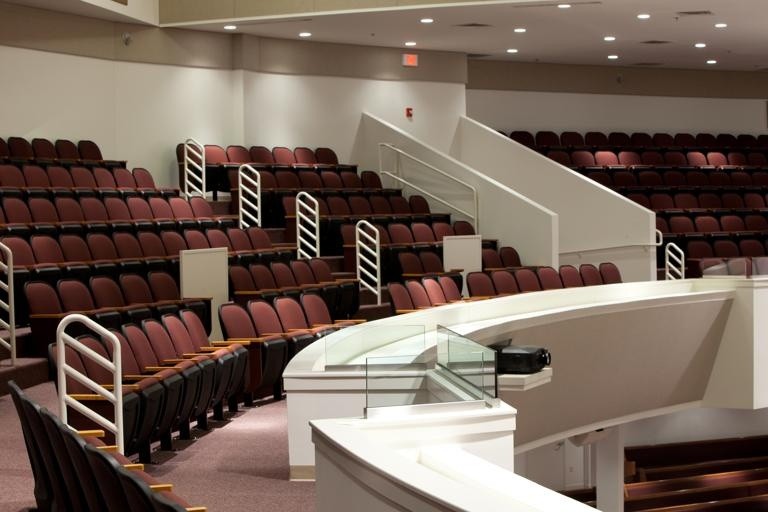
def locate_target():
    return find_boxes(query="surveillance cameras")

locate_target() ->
[122,31,135,47]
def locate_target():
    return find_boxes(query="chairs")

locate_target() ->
[5,377,203,512]
[17,272,395,466]
[2,135,181,279]
[387,261,622,315]
[175,142,526,280]
[494,129,764,275]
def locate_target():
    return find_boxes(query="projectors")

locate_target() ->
[486,337,552,378]
